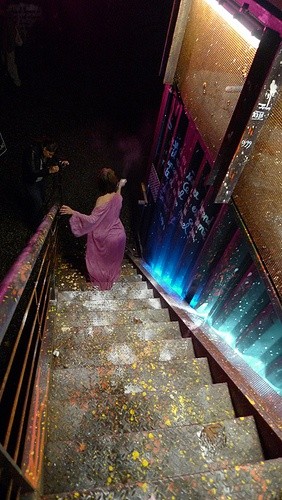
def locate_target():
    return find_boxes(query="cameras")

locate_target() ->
[53,159,72,171]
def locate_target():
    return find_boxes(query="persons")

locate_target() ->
[21,138,69,230]
[58,166,129,292]
